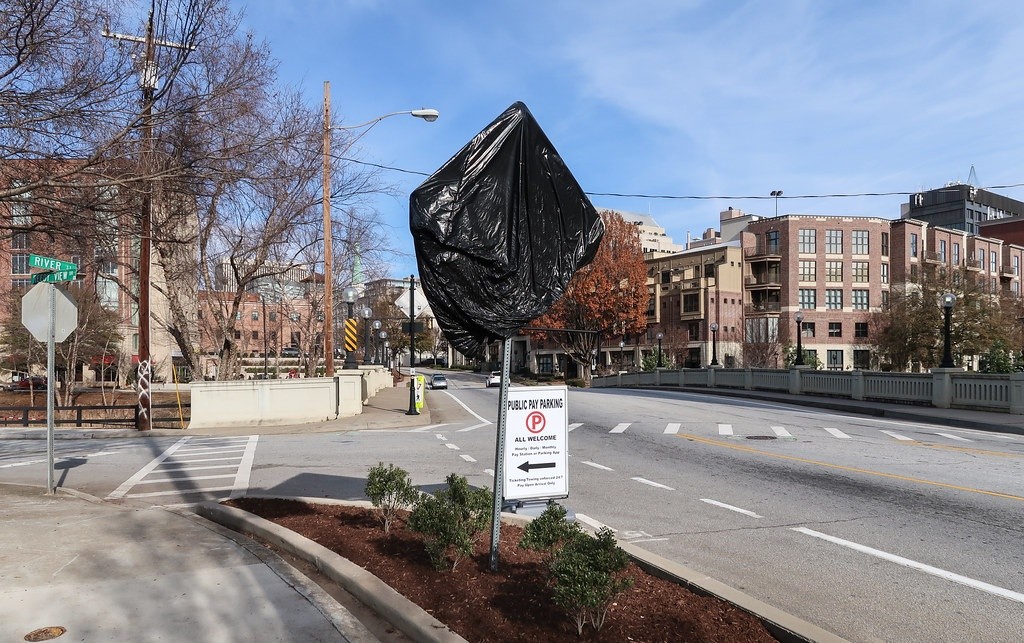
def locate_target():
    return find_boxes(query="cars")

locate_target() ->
[430,372,447,390]
[485,371,510,387]
[18,375,47,390]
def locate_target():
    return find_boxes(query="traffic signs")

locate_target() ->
[28,253,79,286]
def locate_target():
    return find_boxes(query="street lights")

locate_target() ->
[618,341,626,371]
[341,287,392,371]
[710,322,718,365]
[940,293,958,368]
[323,80,440,379]
[656,331,663,367]
[793,311,805,365]
